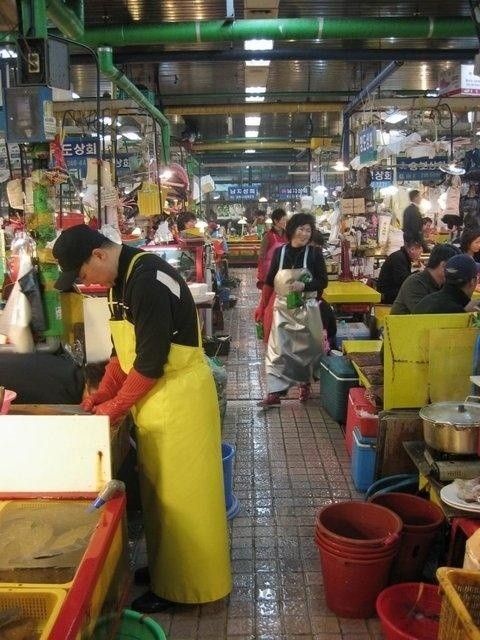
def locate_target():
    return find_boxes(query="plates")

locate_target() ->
[441,482,480,513]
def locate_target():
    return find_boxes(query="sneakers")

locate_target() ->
[297,386,312,401]
[257,393,282,409]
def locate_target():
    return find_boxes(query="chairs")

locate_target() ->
[342,312,477,409]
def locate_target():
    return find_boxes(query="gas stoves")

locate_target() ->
[423,446,480,482]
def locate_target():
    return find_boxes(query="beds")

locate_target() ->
[237,218,247,236]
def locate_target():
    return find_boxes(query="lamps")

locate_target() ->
[330,158,348,171]
[100,117,122,128]
[384,110,407,125]
[121,126,143,140]
[258,195,267,203]
[438,102,467,176]
[314,185,327,195]
[264,211,273,229]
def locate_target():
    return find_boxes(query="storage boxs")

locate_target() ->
[334,320,371,349]
[0,587,67,638]
[351,425,375,494]
[0,499,103,588]
[319,354,360,424]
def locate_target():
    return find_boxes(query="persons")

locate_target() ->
[256,208,289,290]
[51,216,234,614]
[413,253,480,314]
[176,211,198,232]
[422,217,433,233]
[403,190,430,253]
[458,223,480,263]
[380,243,462,366]
[376,238,424,305]
[253,212,328,408]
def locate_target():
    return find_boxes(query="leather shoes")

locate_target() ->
[131,589,195,614]
[133,565,152,585]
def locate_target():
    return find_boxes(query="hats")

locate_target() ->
[52,223,105,292]
[443,253,480,280]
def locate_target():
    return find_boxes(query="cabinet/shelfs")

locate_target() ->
[225,238,260,266]
[145,243,213,341]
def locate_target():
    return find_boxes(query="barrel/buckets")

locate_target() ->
[369,492,443,563]
[314,500,402,619]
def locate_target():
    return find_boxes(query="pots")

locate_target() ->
[419,395,480,454]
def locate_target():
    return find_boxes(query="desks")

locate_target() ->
[9,402,133,480]
[196,299,216,310]
[401,437,479,520]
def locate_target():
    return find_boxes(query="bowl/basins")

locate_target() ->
[189,284,208,293]
[194,292,215,303]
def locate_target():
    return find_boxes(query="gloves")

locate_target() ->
[91,365,158,426]
[254,287,275,323]
[80,356,128,412]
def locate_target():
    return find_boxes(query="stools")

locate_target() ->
[372,303,393,329]
